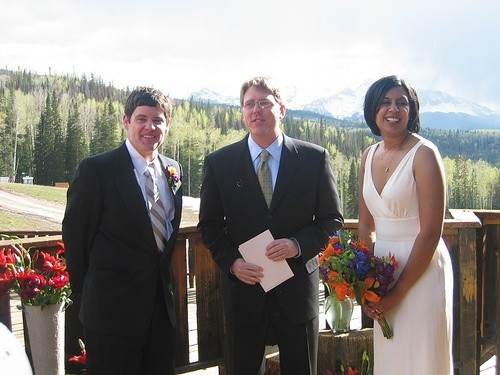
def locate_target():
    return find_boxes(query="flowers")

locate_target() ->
[318,218,399,339]
[69,339,86,365]
[327,351,373,375]
[166,164,179,190]
[0,234,73,311]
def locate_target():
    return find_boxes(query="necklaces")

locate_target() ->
[379,141,401,172]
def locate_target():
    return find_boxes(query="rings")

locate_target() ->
[376,305,384,313]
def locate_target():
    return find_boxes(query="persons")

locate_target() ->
[196,78,345,375]
[357,74,455,375]
[60,87,183,375]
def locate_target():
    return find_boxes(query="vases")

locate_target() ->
[324,282,354,333]
[24,304,65,375]
